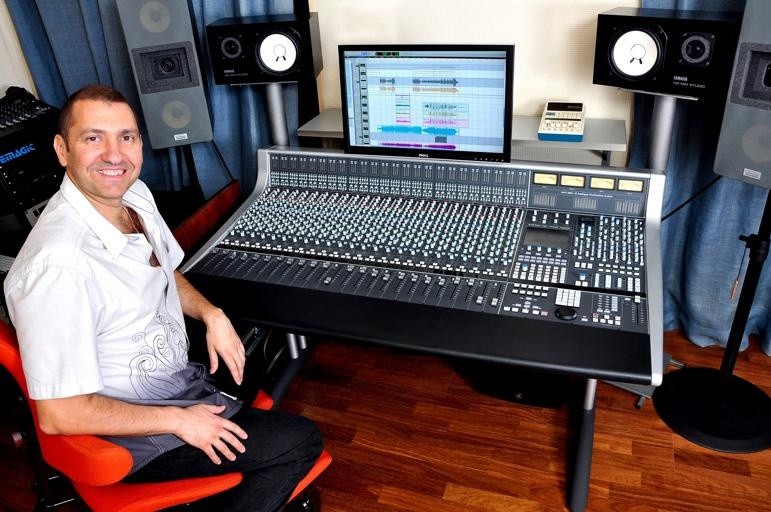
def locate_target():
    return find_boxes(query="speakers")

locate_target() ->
[711,0,771,192]
[591,5,742,102]
[203,11,324,88]
[115,0,215,151]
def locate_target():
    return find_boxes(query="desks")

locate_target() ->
[295,109,625,174]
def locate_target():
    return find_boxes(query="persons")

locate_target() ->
[3,82,328,510]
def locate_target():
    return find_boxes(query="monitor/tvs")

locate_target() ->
[337,43,514,164]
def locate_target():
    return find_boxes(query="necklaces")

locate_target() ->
[122,205,138,233]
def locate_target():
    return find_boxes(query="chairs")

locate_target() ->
[0,324,332,512]
